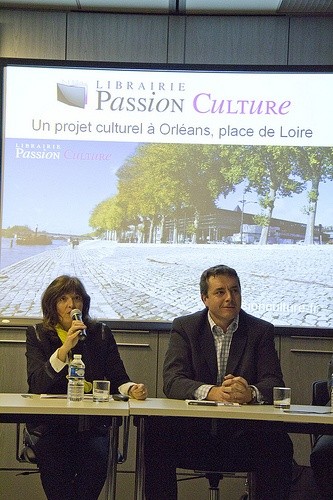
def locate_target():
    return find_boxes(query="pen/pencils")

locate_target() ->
[188,401,217,407]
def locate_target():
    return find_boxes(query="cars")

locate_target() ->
[296,240,304,246]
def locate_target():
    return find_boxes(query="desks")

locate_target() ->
[0,393,333,500]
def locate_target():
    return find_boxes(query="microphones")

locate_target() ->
[70,309,87,340]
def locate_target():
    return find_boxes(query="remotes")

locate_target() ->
[112,394,129,402]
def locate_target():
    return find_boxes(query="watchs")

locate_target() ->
[246,386,256,404]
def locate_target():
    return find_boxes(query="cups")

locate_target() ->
[273,387,291,409]
[92,380,110,403]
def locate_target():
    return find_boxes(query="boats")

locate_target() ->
[15,225,54,246]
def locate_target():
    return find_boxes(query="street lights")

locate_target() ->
[241,201,258,244]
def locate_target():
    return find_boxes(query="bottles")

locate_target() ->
[67,354,85,403]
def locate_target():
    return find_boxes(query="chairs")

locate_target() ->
[14,416,129,500]
[309,380,333,500]
[176,464,252,500]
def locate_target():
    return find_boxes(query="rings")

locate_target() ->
[143,392,145,395]
[69,328,73,333]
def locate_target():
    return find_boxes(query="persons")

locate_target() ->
[143,265,294,500]
[309,355,333,500]
[25,274,147,500]
